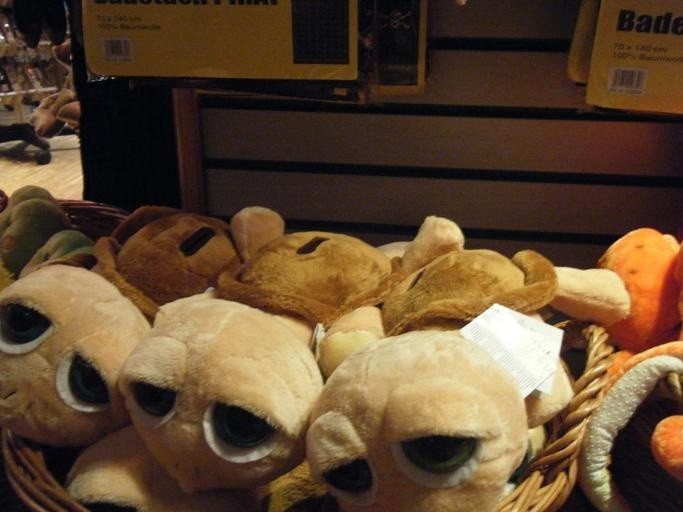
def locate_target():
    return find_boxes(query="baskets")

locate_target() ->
[0,200,614,512]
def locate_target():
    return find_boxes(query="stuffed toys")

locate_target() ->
[0,181,682,511]
[0,182,683,511]
